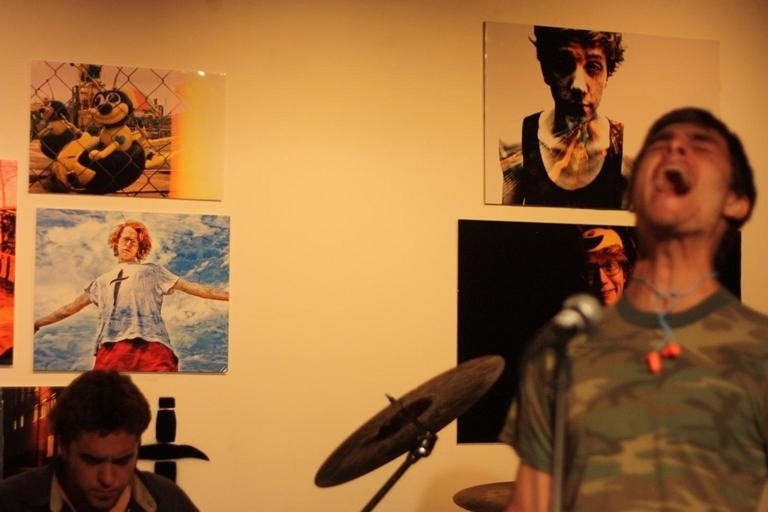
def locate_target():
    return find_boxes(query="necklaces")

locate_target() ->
[629,267,717,374]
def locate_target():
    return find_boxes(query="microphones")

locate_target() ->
[548,292,602,342]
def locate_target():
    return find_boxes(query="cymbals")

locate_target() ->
[314,354,505,487]
[453,481,516,511]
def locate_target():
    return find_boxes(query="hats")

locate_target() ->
[570,227,624,255]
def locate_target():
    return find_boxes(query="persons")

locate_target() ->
[2,370,201,512]
[498,25,638,208]
[499,103,768,512]
[577,228,629,306]
[33,219,230,373]
